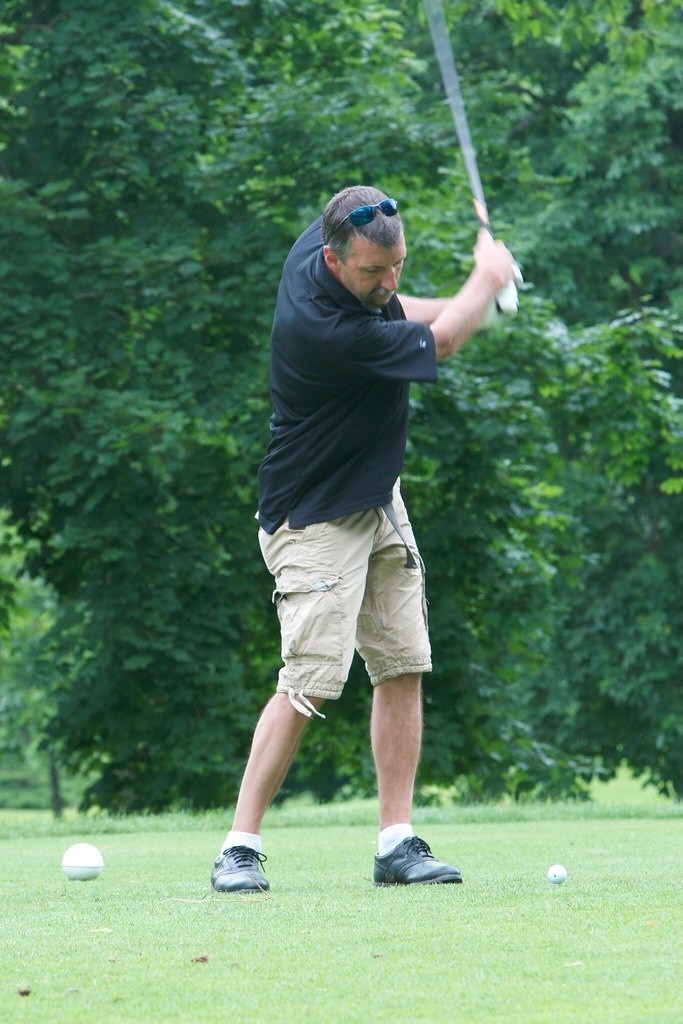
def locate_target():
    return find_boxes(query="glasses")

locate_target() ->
[323,198,400,247]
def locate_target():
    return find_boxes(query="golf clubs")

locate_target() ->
[423,3,520,317]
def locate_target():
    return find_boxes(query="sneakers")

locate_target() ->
[209,854,269,894]
[373,835,463,887]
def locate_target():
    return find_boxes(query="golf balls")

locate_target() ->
[547,865,567,883]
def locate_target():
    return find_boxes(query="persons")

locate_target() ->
[212,181,514,889]
